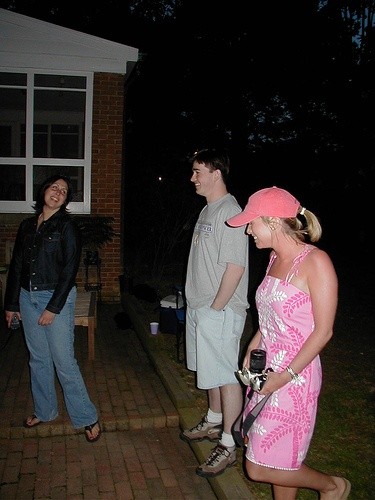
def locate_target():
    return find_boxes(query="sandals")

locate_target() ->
[24,414,42,428]
[85,421,101,442]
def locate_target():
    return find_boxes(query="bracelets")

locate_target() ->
[286,366,296,378]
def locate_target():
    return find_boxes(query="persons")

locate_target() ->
[225,186,351,499]
[4,175,101,442]
[180,149,250,477]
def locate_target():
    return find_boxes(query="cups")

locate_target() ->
[150,322,159,335]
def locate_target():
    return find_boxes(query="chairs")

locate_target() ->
[0,240,15,307]
[177,289,187,364]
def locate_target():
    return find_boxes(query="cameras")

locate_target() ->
[236,348,268,391]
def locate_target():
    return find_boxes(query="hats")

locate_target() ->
[226,186,301,228]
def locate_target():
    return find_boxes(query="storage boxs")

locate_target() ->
[159,294,184,334]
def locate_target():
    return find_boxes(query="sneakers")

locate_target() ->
[181,416,223,442]
[196,442,237,477]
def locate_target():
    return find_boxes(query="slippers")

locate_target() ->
[342,478,351,500]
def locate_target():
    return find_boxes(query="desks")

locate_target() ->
[74,285,98,359]
